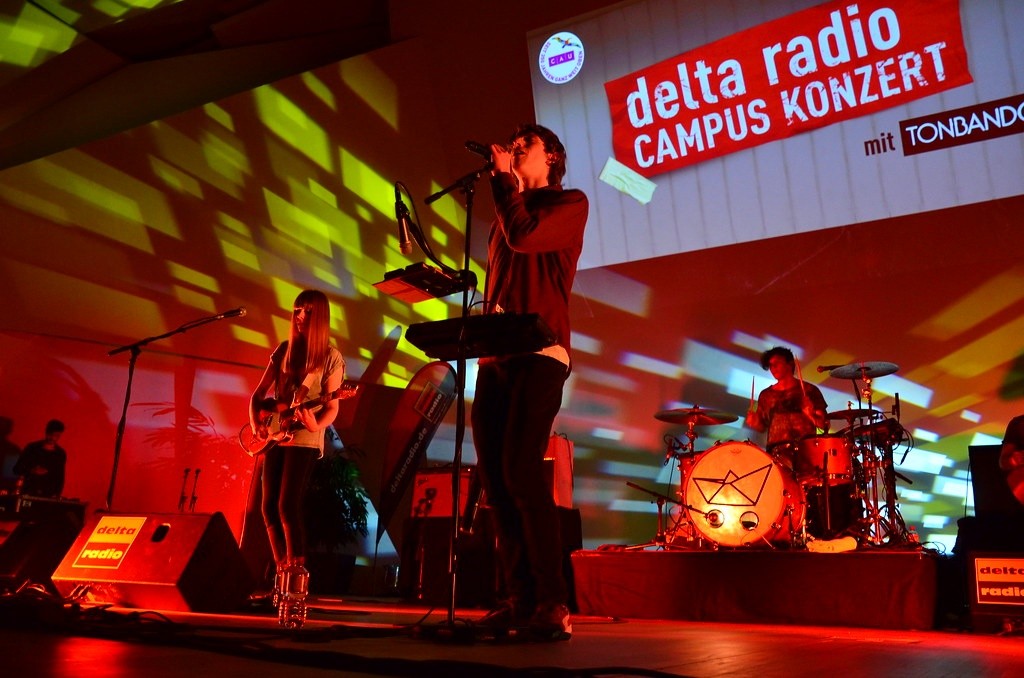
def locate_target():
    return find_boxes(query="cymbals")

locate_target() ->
[653,402,740,427]
[828,359,901,380]
[826,400,880,420]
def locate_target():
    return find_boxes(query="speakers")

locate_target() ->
[410,465,474,518]
[0,507,256,611]
[541,435,574,509]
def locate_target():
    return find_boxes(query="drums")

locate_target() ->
[682,440,808,550]
[797,432,854,487]
[677,450,706,518]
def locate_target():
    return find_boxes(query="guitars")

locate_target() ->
[247,382,360,457]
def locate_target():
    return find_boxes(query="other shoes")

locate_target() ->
[247,588,277,611]
[524,601,573,641]
[475,596,532,629]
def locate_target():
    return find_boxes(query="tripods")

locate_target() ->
[622,482,711,549]
[832,369,916,550]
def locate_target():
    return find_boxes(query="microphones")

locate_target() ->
[465,140,492,161]
[895,393,901,419]
[816,365,840,373]
[707,513,719,522]
[672,436,687,452]
[394,183,413,254]
[214,307,248,320]
[418,489,437,505]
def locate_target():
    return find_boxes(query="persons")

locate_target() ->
[1003,415,1024,631]
[471,124,589,642]
[747,347,829,546]
[14,419,67,522]
[250,289,345,605]
